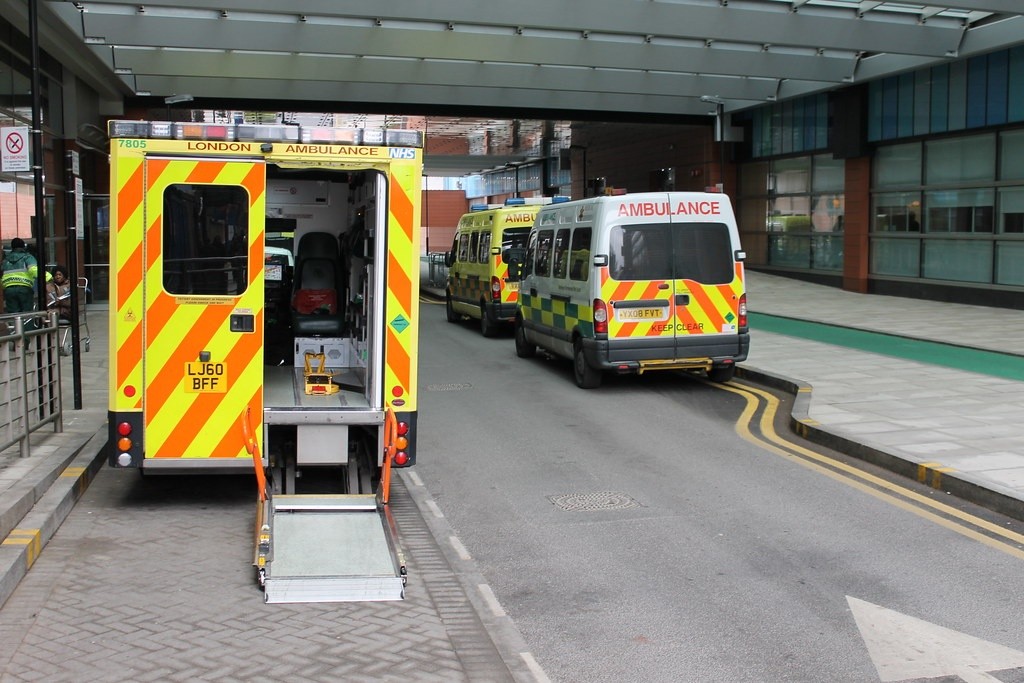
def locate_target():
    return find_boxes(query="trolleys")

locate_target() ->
[46,276,92,357]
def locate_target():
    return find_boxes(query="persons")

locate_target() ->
[45,265,73,321]
[0,238,54,352]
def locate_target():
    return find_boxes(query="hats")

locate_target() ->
[52,266,68,279]
[11,238,25,250]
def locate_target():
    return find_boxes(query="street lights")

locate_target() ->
[504,163,521,198]
[162,93,196,121]
[569,143,588,199]
[701,95,726,193]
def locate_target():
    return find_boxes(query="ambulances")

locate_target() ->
[104,116,426,606]
[501,182,752,389]
[441,195,572,339]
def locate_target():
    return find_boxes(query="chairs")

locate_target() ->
[291,232,346,338]
